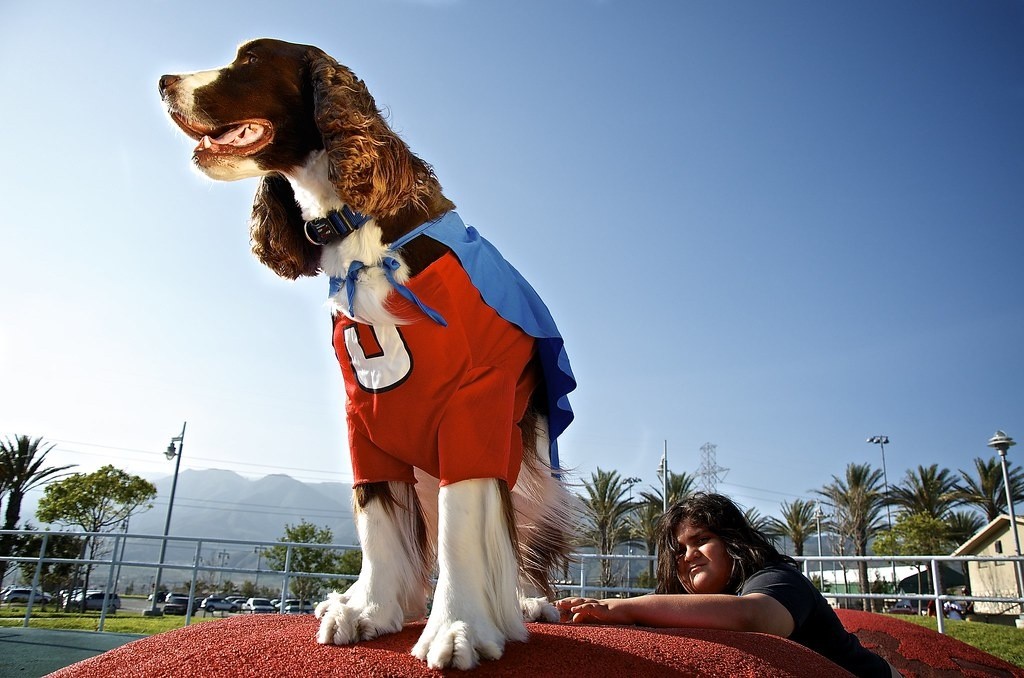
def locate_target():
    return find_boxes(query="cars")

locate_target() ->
[195,596,313,613]
[147,591,197,617]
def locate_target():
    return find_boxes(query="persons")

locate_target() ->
[553,494,895,678]
[927,594,964,621]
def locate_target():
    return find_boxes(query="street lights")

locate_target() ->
[657,439,670,523]
[812,497,825,593]
[142,421,188,616]
[986,430,1024,629]
[866,435,898,593]
[622,477,641,594]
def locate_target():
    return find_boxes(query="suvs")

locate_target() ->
[201,597,237,615]
[0,585,51,604]
[60,586,121,610]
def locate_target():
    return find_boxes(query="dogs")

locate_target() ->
[159,36,604,673]
